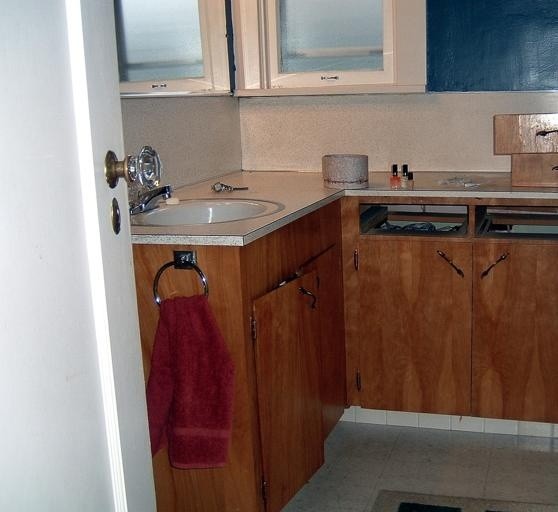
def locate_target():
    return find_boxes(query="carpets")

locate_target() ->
[369,489,558,512]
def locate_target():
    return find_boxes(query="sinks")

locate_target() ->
[133,198,284,225]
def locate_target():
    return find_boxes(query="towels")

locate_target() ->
[147,294,235,470]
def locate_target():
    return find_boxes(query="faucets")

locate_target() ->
[129,183,171,213]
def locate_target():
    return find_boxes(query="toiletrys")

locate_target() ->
[390,164,400,190]
[401,163,408,188]
[407,172,414,189]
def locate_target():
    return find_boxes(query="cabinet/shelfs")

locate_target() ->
[114,0,426,97]
[341,191,558,423]
[133,199,347,512]
